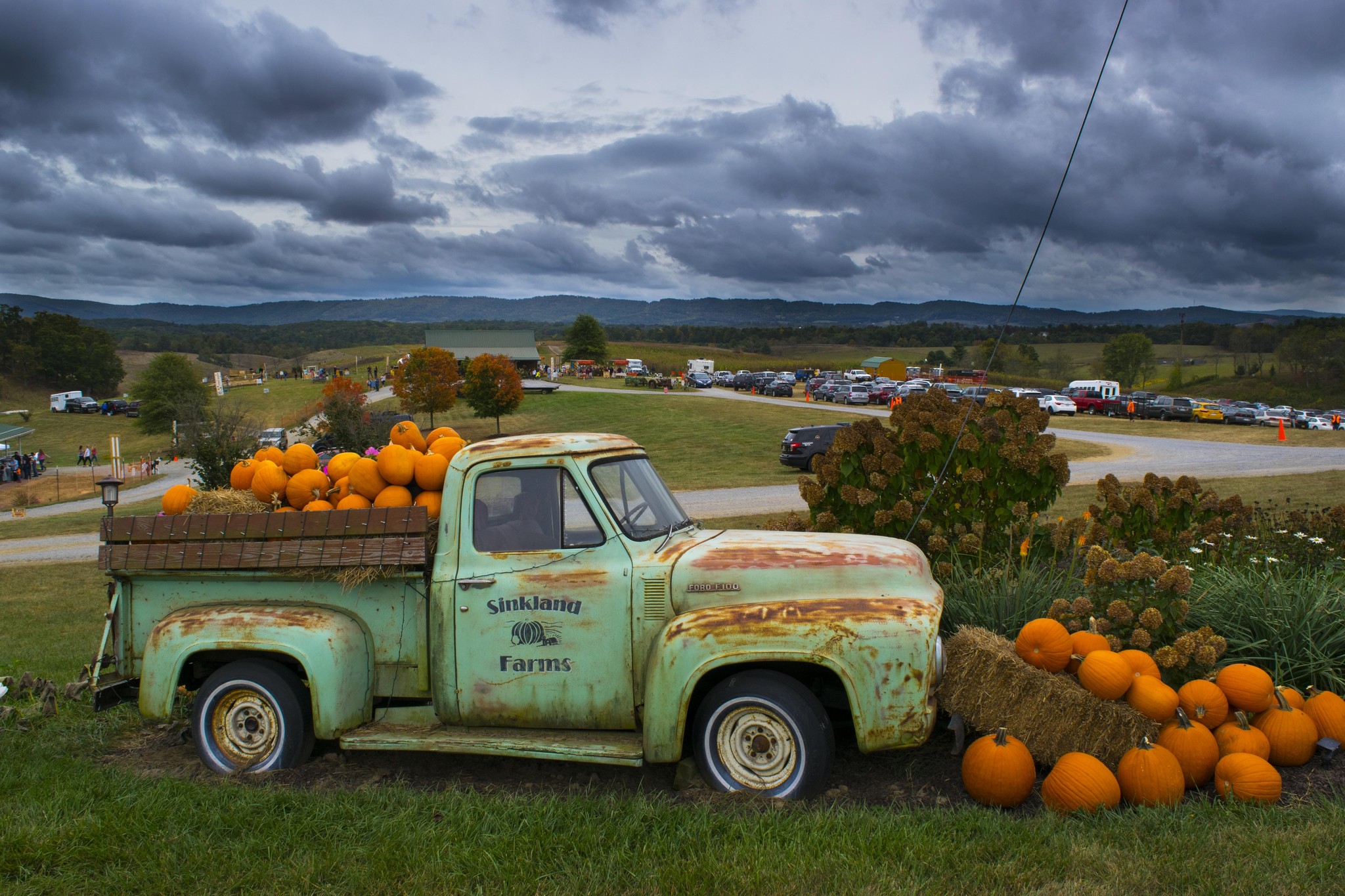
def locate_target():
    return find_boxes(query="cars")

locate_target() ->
[1190,401,1224,424]
[687,372,713,389]
[763,380,793,397]
[713,363,1345,433]
[316,449,348,472]
[310,431,345,454]
[1219,406,1256,426]
[813,383,842,402]
[832,384,869,406]
[719,374,736,387]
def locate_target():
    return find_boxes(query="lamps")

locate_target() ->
[95,474,124,517]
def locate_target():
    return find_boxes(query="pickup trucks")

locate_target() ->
[844,369,872,383]
[1066,389,1121,416]
[66,396,101,414]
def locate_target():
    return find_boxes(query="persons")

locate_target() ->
[0,444,100,483]
[1331,412,1341,430]
[1287,408,1298,428]
[1127,397,1134,423]
[874,368,919,380]
[523,363,656,380]
[204,365,386,393]
[1235,404,1239,408]
[805,367,853,397]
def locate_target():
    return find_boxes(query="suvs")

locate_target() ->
[99,399,128,416]
[779,422,853,474]
[732,375,757,391]
[793,369,815,382]
[1144,397,1193,422]
[124,399,151,417]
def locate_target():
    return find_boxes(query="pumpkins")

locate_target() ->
[961,727,1036,809]
[1065,616,1111,677]
[161,478,200,515]
[1115,649,1161,680]
[1115,736,1184,809]
[230,421,471,520]
[1070,650,1133,700]
[1124,675,1180,722]
[1041,752,1120,816]
[1155,664,1345,808]
[1015,618,1073,673]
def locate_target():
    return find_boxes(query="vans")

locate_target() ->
[256,426,288,450]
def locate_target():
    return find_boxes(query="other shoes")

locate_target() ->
[44,467,47,471]
[98,465,101,466]
[30,477,32,479]
[25,478,29,479]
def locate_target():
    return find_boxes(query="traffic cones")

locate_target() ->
[752,386,755,394]
[806,391,809,402]
[889,397,894,410]
[1276,418,1289,441]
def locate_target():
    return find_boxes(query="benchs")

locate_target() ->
[472,491,558,552]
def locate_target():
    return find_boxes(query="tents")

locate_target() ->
[0,444,10,457]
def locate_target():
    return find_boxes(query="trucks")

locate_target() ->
[67,428,952,808]
[625,359,647,376]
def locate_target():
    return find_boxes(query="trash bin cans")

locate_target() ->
[339,370,343,376]
[332,375,337,378]
